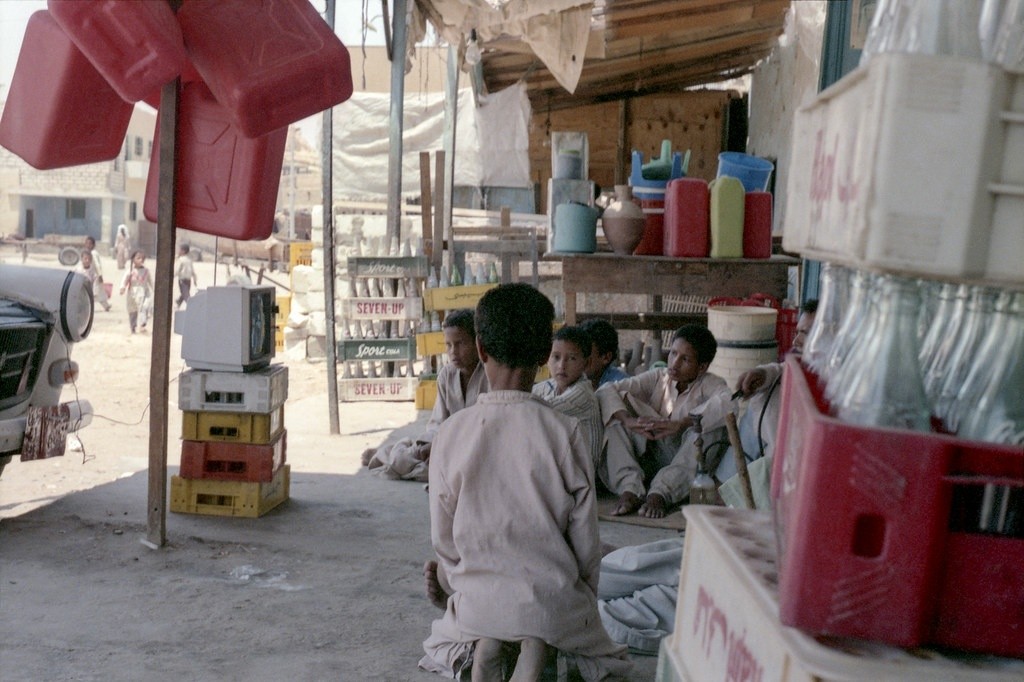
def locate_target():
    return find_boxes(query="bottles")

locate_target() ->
[340,318,417,340]
[346,276,421,299]
[801,265,1024,539]
[427,261,499,290]
[422,309,441,332]
[364,234,423,257]
[343,361,415,379]
[858,0,1021,79]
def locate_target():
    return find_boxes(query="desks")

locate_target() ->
[539,247,798,402]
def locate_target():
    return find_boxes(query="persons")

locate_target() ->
[594,324,740,521]
[425,283,601,682]
[117,250,154,335]
[83,235,112,312]
[173,243,198,310]
[73,249,97,289]
[529,325,604,467]
[425,298,821,654]
[363,306,489,483]
[582,319,627,392]
[113,224,132,269]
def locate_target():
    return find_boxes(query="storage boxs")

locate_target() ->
[328,253,507,411]
[650,47,1024,682]
[167,365,293,518]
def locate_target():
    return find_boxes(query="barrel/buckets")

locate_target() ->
[717,151,774,192]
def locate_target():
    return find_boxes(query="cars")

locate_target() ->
[0,261,92,473]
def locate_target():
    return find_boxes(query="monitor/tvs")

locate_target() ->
[175,284,278,372]
